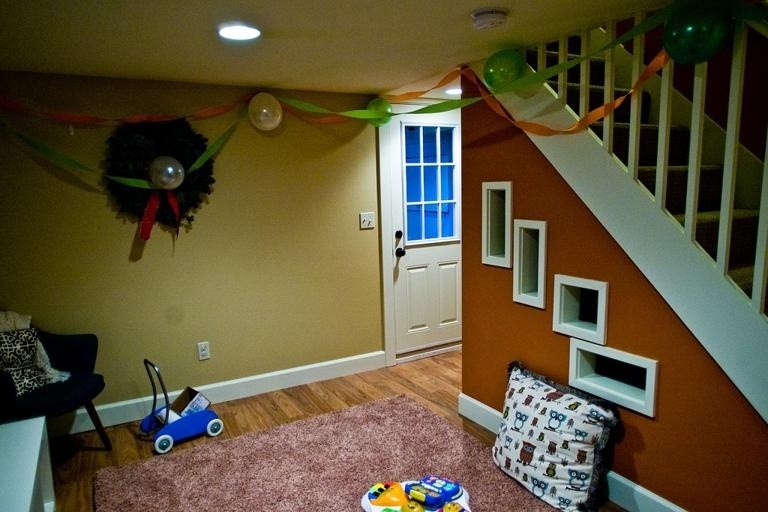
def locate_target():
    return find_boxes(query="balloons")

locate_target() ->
[247,93,283,131]
[483,50,525,90]
[366,98,392,127]
[149,155,185,190]
[662,0,728,66]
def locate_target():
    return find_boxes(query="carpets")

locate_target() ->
[91,392,562,510]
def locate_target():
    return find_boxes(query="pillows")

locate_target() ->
[492,361,623,511]
[1,326,50,397]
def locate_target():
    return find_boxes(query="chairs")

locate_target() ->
[0,325,113,453]
